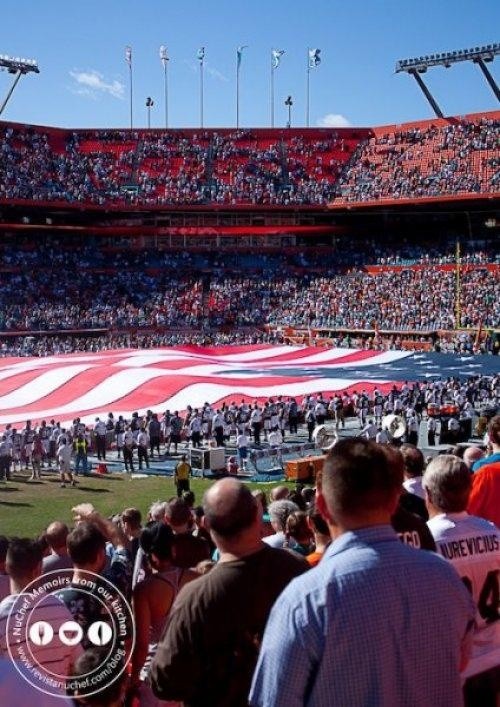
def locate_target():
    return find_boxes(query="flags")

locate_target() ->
[197,46,204,65]
[159,44,169,64]
[272,49,286,70]
[308,48,321,70]
[236,45,248,70]
[124,46,132,66]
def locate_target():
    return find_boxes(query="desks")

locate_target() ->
[282,456,326,483]
[191,447,225,477]
